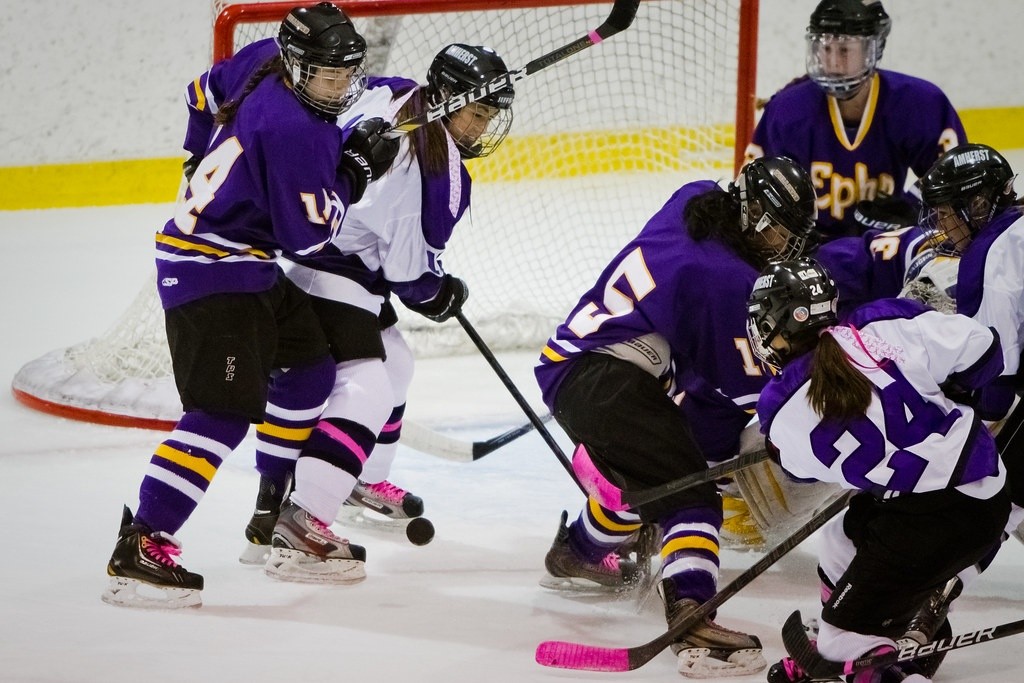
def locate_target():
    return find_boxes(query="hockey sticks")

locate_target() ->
[534,489,861,674]
[571,443,770,513]
[397,413,555,466]
[379,1,643,141]
[452,308,587,499]
[780,609,1024,680]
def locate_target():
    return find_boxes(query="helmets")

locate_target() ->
[427,44,515,158]
[728,155,819,263]
[805,0,893,101]
[745,257,839,376]
[914,143,1018,257]
[274,1,368,115]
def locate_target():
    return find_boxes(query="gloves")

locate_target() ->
[399,273,469,323]
[937,378,981,405]
[339,117,401,205]
[181,156,202,183]
[848,199,920,237]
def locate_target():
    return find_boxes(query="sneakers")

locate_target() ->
[655,579,767,679]
[264,501,368,585]
[100,505,204,609]
[537,510,639,593]
[846,646,934,683]
[335,480,425,532]
[239,475,368,564]
[767,563,837,683]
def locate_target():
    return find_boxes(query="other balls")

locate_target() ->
[406,518,436,547]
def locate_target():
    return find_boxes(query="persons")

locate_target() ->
[263,42,515,586]
[534,1,1024,683]
[102,0,400,609]
